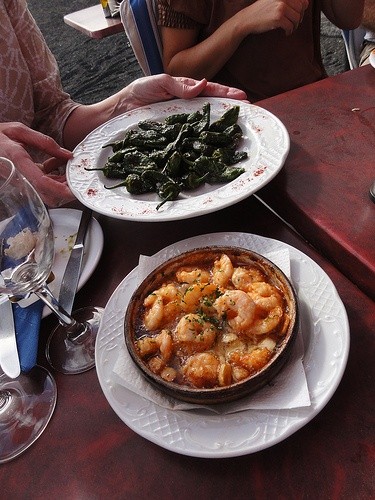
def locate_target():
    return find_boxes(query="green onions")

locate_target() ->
[176,270,235,335]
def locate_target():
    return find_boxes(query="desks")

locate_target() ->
[0,64,375,500]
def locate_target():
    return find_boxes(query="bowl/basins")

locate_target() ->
[123,246,298,403]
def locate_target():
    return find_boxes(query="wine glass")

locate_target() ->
[0,363,59,464]
[0,157,105,375]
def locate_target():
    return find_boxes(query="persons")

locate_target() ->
[0,0,247,207]
[158,0,365,103]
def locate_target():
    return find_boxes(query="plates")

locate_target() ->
[65,96,290,222]
[95,232,351,458]
[0,208,104,319]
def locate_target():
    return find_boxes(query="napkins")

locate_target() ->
[0,201,43,373]
[109,247,311,415]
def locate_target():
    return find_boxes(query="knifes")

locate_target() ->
[57,205,92,326]
[0,277,21,379]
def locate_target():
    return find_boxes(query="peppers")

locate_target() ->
[84,102,248,210]
